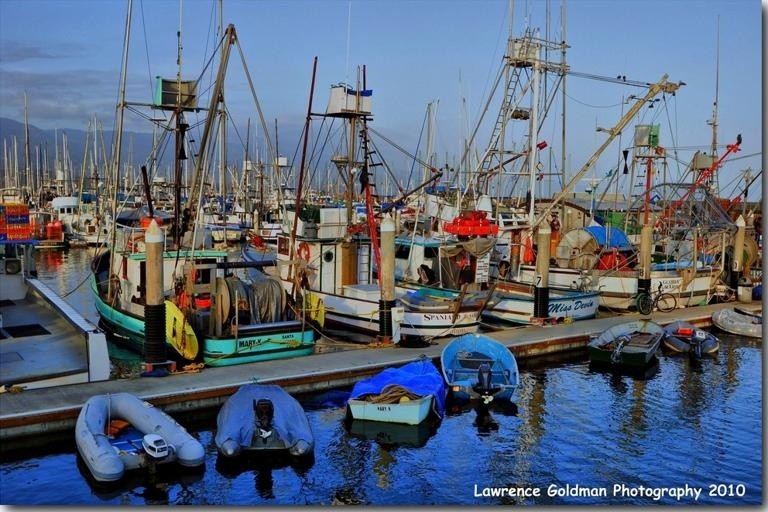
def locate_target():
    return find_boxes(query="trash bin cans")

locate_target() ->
[738,277,754,304]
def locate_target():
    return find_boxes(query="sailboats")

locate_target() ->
[2,0,761,484]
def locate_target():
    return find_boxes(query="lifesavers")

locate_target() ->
[296,244,309,261]
[450,252,466,266]
